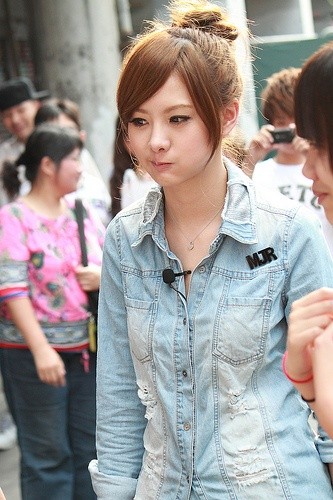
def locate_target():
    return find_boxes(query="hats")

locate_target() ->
[0,79,50,112]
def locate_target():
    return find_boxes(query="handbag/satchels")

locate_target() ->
[75,198,99,324]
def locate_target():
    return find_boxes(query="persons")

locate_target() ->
[88,0,333,500]
[301,394,316,403]
[0,79,158,500]
[224,43,333,489]
[283,351,313,384]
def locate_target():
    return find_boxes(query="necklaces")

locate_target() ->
[164,202,224,250]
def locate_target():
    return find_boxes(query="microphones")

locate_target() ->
[163,269,175,284]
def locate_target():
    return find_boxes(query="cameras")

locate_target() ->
[270,127,295,144]
